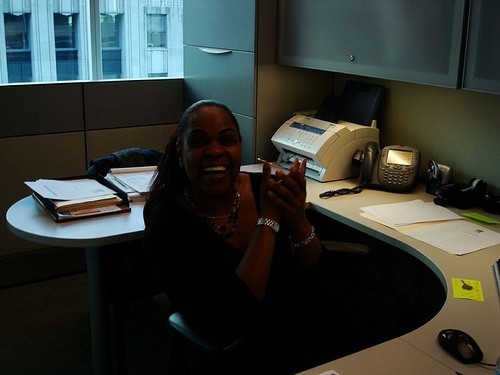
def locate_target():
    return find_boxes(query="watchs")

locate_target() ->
[255,217,282,233]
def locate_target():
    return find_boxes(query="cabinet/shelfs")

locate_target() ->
[275,0,500,94]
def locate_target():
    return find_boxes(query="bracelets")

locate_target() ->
[289,226,315,254]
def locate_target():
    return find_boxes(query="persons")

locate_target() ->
[143,101,321,375]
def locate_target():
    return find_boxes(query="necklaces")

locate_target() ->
[185,183,241,239]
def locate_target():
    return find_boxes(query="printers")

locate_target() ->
[272,80,386,182]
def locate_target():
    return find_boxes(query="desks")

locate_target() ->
[6,161,500,375]
